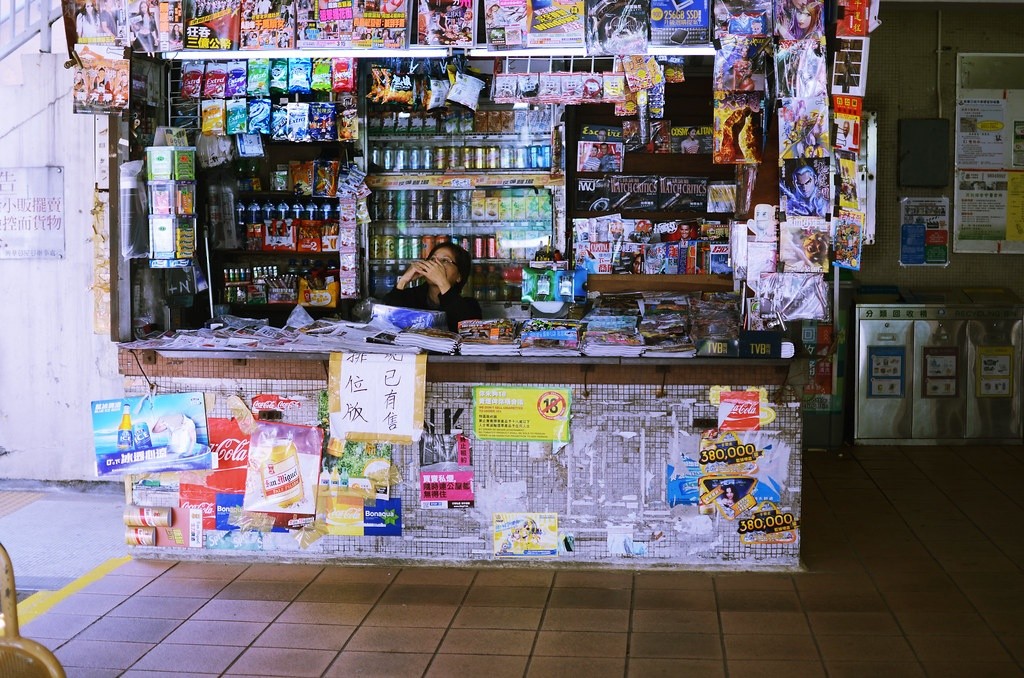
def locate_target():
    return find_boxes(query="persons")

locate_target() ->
[680,126,700,155]
[383,241,484,334]
[836,122,858,151]
[632,254,641,275]
[677,223,697,241]
[581,143,618,172]
[597,129,608,142]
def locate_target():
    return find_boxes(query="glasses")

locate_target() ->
[430,255,456,267]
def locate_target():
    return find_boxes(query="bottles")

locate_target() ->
[341,468,349,485]
[234,198,340,222]
[331,467,340,487]
[207,184,222,248]
[319,466,330,487]
[372,265,410,299]
[286,258,340,280]
[118,404,133,453]
[474,265,520,301]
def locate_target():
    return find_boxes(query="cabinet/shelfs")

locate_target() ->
[168,60,734,321]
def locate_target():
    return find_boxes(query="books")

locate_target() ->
[572,174,733,275]
[736,159,865,332]
[74,0,873,167]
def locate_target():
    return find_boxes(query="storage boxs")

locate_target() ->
[698,335,740,358]
[741,325,781,358]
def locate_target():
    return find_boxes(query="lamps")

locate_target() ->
[464,44,717,56]
[162,45,453,59]
